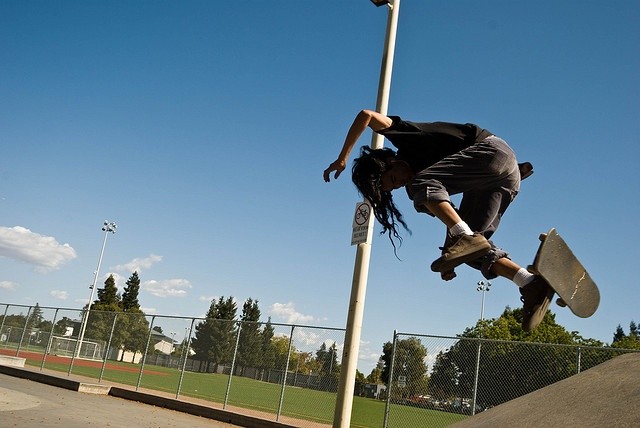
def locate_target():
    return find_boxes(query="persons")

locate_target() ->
[324,108,555,333]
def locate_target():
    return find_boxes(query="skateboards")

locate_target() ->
[526,227,601,318]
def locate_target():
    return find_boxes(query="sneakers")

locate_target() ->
[519,275,554,333]
[429,230,491,272]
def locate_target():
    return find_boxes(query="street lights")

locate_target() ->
[472,281,491,415]
[177,328,189,370]
[76,220,117,357]
[169,331,177,367]
[293,350,303,386]
